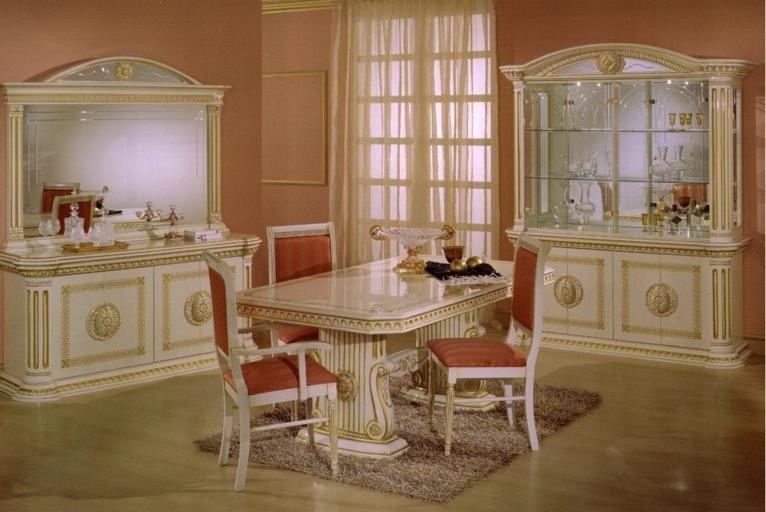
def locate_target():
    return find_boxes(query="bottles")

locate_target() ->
[547,180,596,224]
[648,144,691,181]
[64,202,115,248]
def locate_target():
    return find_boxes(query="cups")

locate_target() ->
[442,245,464,262]
[441,284,466,300]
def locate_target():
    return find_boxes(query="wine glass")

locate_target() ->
[38,217,61,247]
[668,112,704,132]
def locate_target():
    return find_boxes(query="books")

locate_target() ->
[186,228,219,242]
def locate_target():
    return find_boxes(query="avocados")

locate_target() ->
[450,259,467,272]
[465,256,483,269]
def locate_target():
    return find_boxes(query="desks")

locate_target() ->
[234,255,555,459]
[23,208,184,238]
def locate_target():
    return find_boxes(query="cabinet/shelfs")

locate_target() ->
[498,42,759,368]
[0,231,263,402]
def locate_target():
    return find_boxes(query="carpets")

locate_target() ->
[191,369,602,502]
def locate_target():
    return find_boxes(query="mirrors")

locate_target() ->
[0,56,231,240]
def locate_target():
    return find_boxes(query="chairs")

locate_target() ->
[598,182,612,221]
[51,193,94,235]
[266,221,337,408]
[202,249,339,491]
[40,182,80,215]
[428,233,552,456]
[671,183,707,208]
